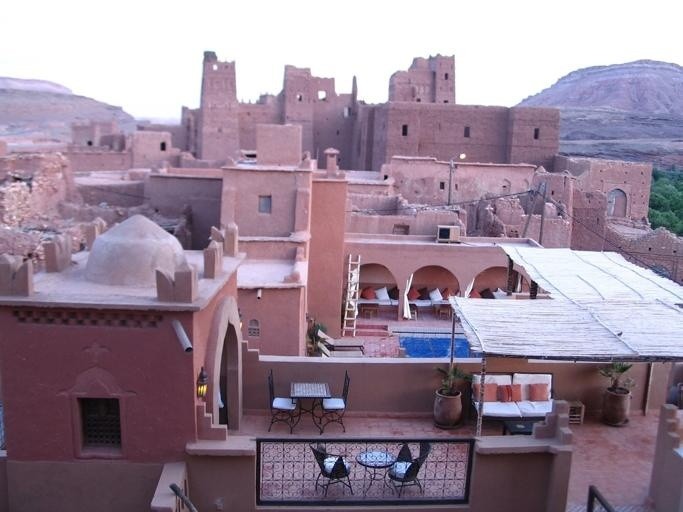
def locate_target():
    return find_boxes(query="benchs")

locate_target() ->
[467,371,559,426]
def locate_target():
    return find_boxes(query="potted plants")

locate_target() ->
[432,363,477,429]
[598,360,639,426]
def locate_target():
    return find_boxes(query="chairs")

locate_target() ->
[316,329,365,358]
[268,369,351,436]
[308,439,432,499]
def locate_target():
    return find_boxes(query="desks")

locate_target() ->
[503,421,538,435]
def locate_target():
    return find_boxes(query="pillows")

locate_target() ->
[472,383,550,403]
[349,285,508,302]
